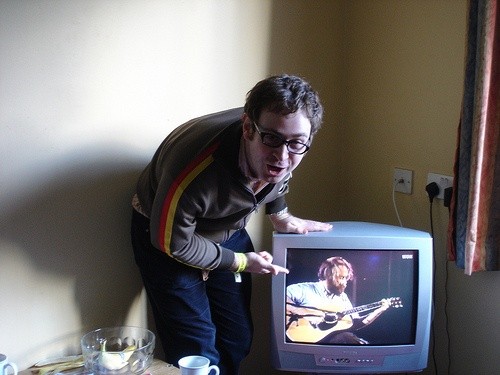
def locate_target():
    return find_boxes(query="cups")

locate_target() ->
[179,356,220,375]
[0,353,18,375]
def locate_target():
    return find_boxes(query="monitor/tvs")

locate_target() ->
[272,221,434,375]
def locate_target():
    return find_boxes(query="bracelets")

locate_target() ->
[232,252,248,282]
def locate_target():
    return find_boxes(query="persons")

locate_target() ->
[285,256,394,346]
[130,73,333,375]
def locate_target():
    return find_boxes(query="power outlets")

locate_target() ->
[426,172,454,199]
[392,168,414,195]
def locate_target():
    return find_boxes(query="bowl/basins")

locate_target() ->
[82,327,155,375]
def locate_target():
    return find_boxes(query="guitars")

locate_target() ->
[285,297,404,343]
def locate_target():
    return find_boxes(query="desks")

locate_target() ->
[8,343,181,375]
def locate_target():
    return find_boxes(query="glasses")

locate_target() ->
[253,120,312,154]
